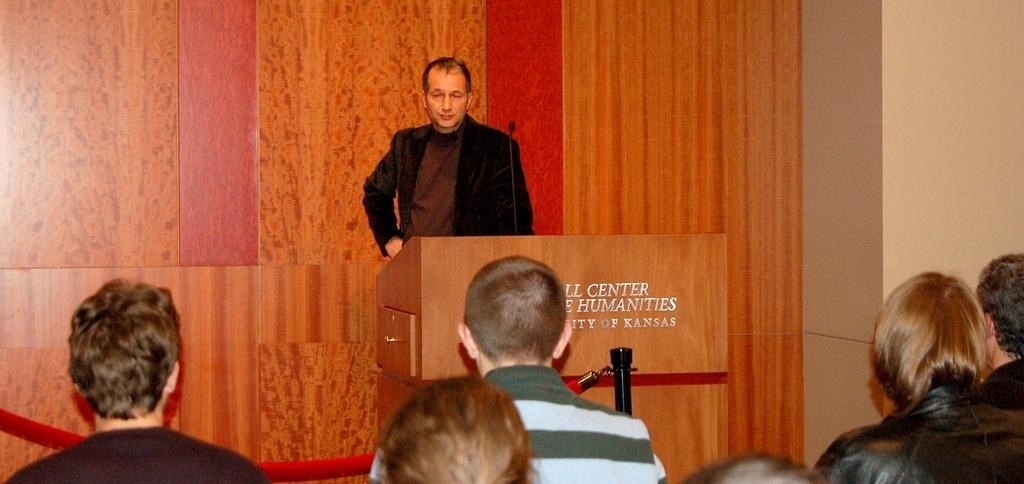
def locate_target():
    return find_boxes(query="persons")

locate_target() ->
[378,374,540,484]
[976,252,1024,410]
[4,278,274,483]
[361,57,532,260]
[683,452,828,483]
[366,256,666,482]
[810,273,1023,483]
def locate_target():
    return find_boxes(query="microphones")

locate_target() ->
[508,121,517,235]
